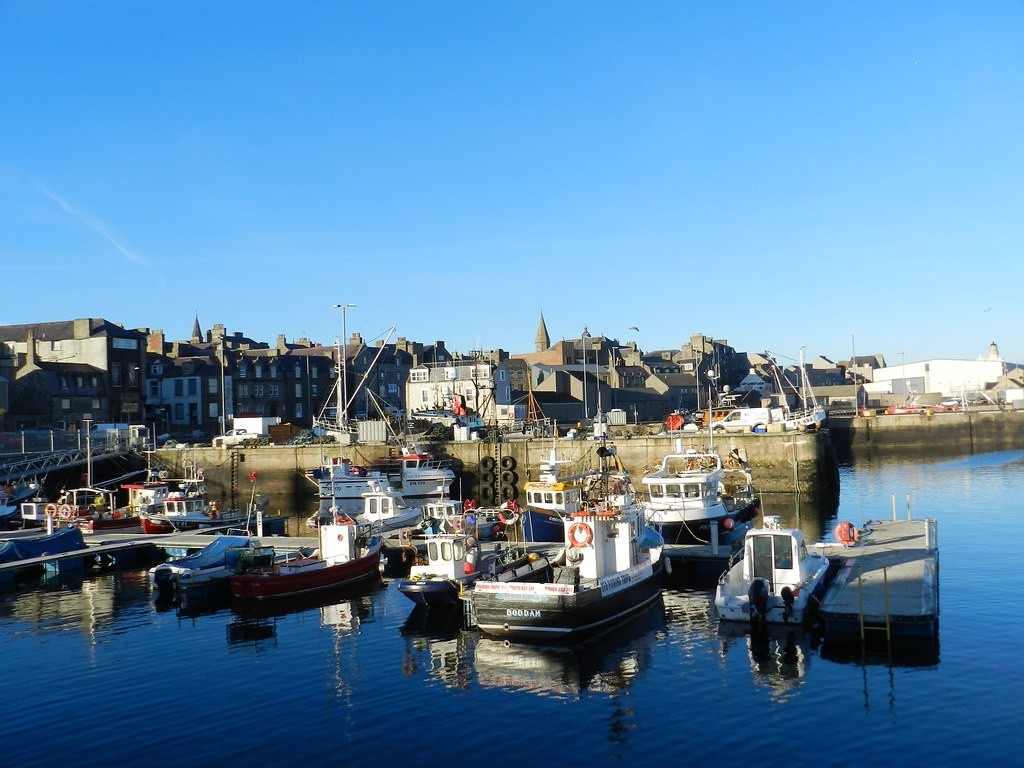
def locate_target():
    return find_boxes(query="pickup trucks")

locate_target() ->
[212,428,260,447]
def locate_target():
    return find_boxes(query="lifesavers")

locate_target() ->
[500,456,513,470]
[570,511,596,516]
[598,510,620,516]
[498,502,519,525]
[478,485,494,503]
[44,503,57,519]
[479,471,496,485]
[499,471,515,485]
[501,485,515,503]
[334,515,352,525]
[834,522,860,547]
[481,456,495,471]
[60,504,72,519]
[567,523,593,547]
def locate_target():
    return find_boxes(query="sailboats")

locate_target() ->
[312,325,399,446]
[764,348,828,432]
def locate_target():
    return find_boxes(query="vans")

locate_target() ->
[710,406,772,434]
[82,423,129,442]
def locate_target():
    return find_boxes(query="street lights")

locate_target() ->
[851,333,859,416]
[579,330,591,420]
[594,345,600,393]
[333,304,357,430]
[611,346,619,409]
[897,352,906,406]
[127,366,140,426]
[693,348,700,411]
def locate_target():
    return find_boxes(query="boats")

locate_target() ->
[519,417,639,543]
[687,363,751,427]
[465,390,666,640]
[0,387,551,613]
[640,384,759,539]
[712,486,830,627]
